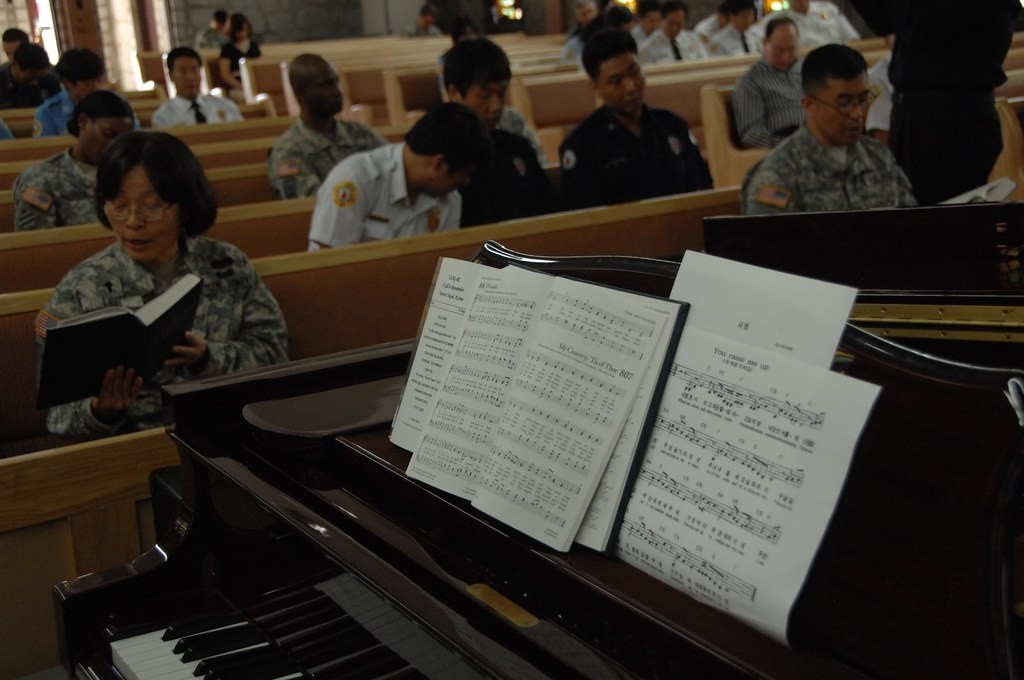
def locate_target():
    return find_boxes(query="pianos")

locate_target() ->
[50,239,1022,678]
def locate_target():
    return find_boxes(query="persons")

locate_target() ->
[775,0,859,48]
[33,48,141,138]
[405,5,441,38]
[731,15,805,147]
[309,101,494,251]
[740,43,919,215]
[497,104,545,164]
[562,0,599,41]
[194,10,229,47]
[559,29,714,209]
[151,47,245,128]
[628,0,709,66]
[0,28,55,73]
[440,36,566,230]
[268,55,392,200]
[559,5,634,59]
[693,0,765,56]
[32,131,289,435]
[218,13,261,89]
[13,92,135,231]
[0,114,15,140]
[0,42,62,109]
[852,0,1024,203]
[405,262,690,553]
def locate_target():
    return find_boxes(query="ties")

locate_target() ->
[741,32,750,53]
[671,37,682,61]
[191,101,207,124]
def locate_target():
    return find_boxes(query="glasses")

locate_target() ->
[804,89,877,119]
[103,194,175,222]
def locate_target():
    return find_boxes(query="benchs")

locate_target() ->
[0,32,1024,458]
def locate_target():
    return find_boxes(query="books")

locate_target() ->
[35,272,203,407]
